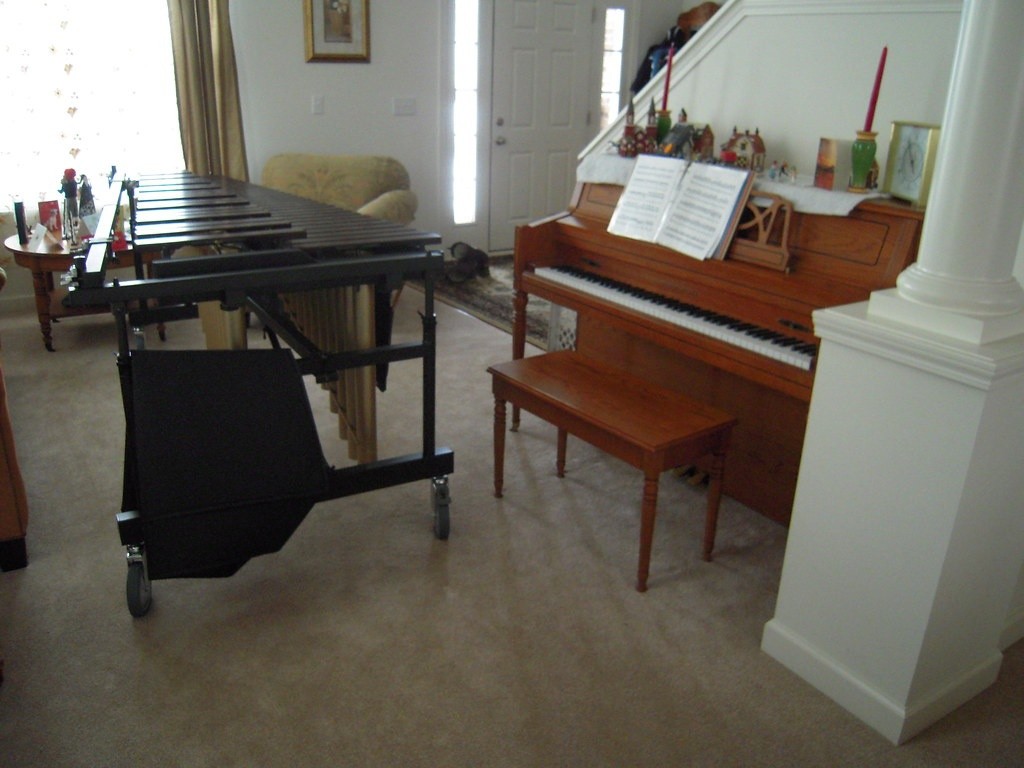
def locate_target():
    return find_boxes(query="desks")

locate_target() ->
[4,235,170,352]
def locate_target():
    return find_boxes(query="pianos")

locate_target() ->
[510,151,926,529]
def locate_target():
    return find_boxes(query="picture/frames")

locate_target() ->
[303,0,374,66]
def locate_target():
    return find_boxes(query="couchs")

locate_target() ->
[263,151,419,228]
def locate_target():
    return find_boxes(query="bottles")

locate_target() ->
[77,174,95,226]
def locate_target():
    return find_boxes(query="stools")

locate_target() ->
[480,351,735,591]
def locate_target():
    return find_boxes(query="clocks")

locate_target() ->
[880,122,941,208]
